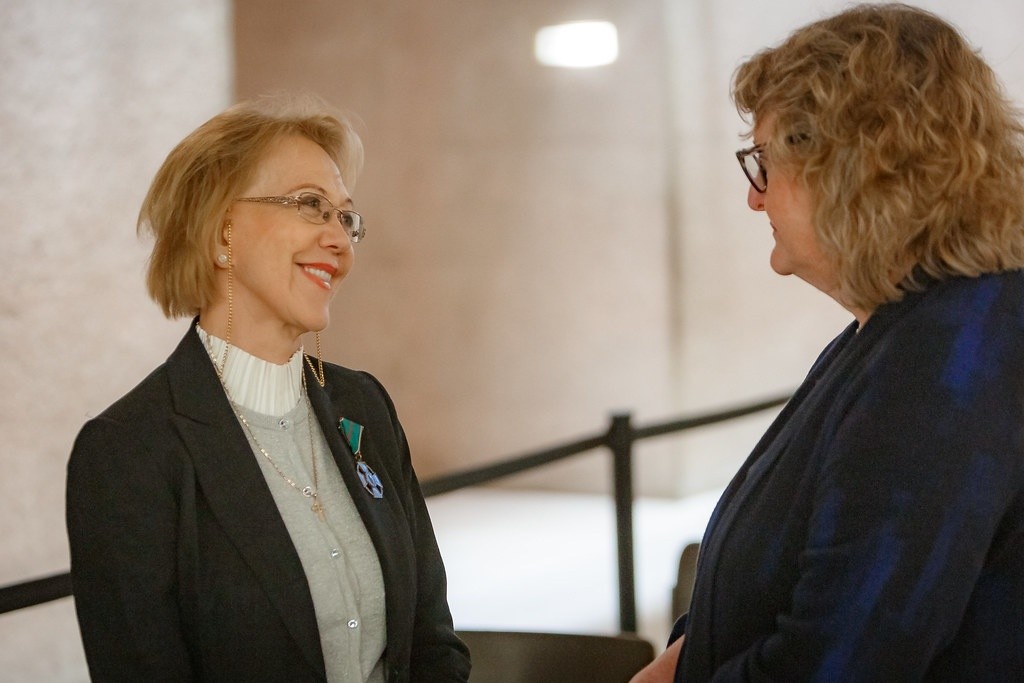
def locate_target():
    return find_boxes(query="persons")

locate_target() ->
[64,93,476,683]
[628,1,1024,683]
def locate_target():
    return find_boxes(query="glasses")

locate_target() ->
[232,191,367,243]
[735,132,809,194]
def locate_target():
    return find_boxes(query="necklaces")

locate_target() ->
[197,322,327,518]
